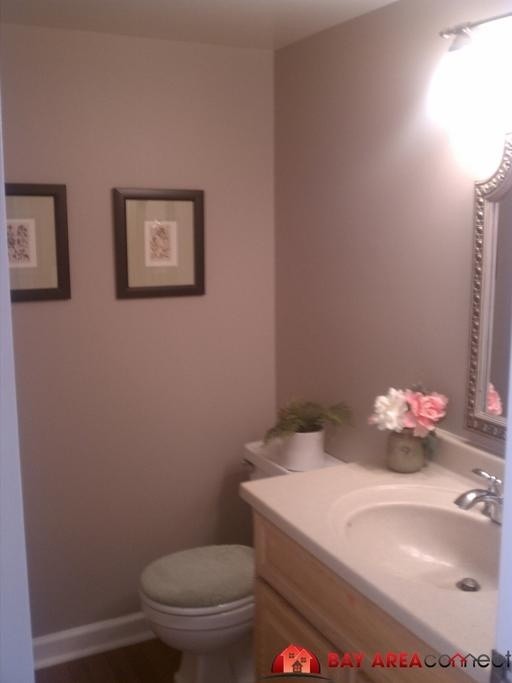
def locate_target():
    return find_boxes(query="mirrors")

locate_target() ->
[463,130,512,442]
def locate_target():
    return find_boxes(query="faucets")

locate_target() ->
[453,468,504,525]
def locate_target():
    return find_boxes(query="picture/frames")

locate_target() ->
[6,181,70,306]
[110,184,205,299]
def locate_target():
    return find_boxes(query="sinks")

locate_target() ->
[342,500,500,595]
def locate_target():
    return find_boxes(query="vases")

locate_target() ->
[381,428,425,474]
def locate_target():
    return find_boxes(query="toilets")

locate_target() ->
[136,437,348,683]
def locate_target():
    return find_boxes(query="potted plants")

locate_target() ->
[262,402,350,473]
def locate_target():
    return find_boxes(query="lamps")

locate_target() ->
[435,10,512,60]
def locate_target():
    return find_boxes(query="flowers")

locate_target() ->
[370,386,450,439]
[486,386,503,414]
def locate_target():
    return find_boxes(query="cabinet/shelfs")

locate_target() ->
[254,512,479,682]
